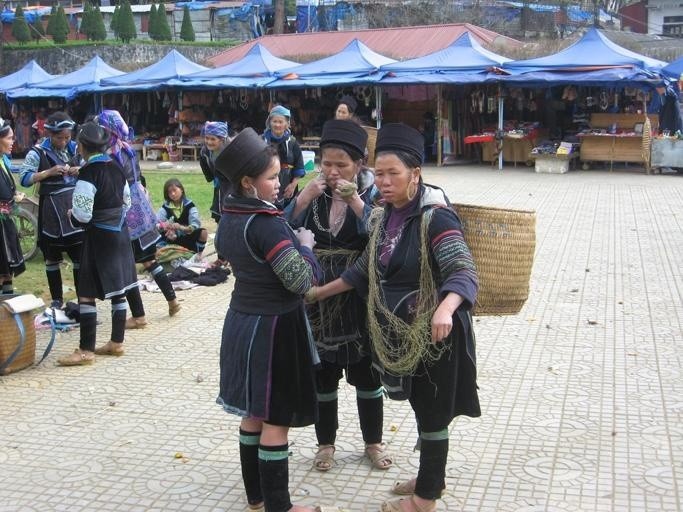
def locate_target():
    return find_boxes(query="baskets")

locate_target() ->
[360,126,378,168]
[0,294,36,375]
[452,203,536,315]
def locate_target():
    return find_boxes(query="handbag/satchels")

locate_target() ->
[125,182,156,241]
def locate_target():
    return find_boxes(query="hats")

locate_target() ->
[43,113,75,132]
[339,96,357,112]
[78,122,110,146]
[0,118,10,136]
[376,123,423,163]
[320,120,367,154]
[215,127,269,182]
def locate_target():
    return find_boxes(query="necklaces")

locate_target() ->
[312,197,349,234]
[377,216,407,247]
[323,189,367,200]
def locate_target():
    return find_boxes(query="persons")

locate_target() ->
[304,122,483,510]
[280,119,395,473]
[334,95,369,166]
[257,106,306,212]
[212,127,323,512]
[417,111,435,145]
[158,180,207,251]
[199,120,237,270]
[1,109,182,368]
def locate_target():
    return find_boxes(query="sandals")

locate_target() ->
[96,341,123,356]
[60,349,93,365]
[391,479,416,494]
[313,444,335,470]
[169,302,181,316]
[125,318,146,329]
[364,442,393,468]
[381,494,436,512]
[317,505,347,511]
